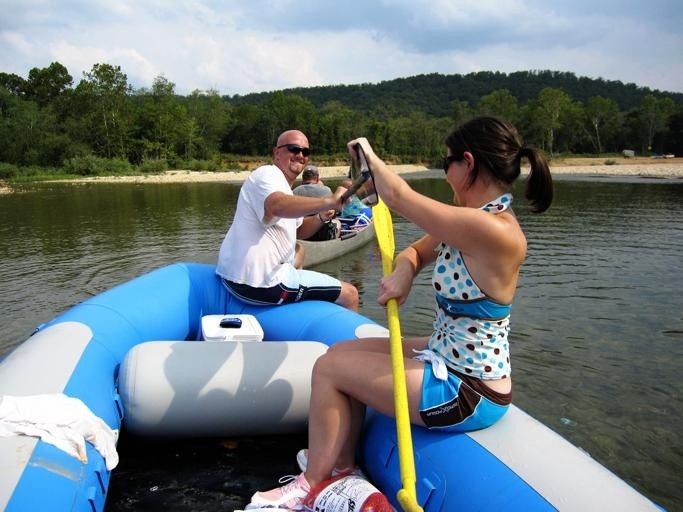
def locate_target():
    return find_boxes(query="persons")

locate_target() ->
[290,164,342,242]
[246,112,528,511]
[214,129,362,314]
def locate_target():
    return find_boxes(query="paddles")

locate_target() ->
[372,197,423,507]
[342,143,378,207]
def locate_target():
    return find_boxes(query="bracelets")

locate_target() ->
[318,213,325,224]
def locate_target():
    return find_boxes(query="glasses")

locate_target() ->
[443,155,455,175]
[277,144,311,156]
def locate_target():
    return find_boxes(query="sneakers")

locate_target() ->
[296,449,359,477]
[251,471,311,510]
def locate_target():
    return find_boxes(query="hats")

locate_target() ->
[303,165,318,175]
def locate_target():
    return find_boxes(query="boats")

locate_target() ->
[295,222,375,268]
[1,260,668,512]
